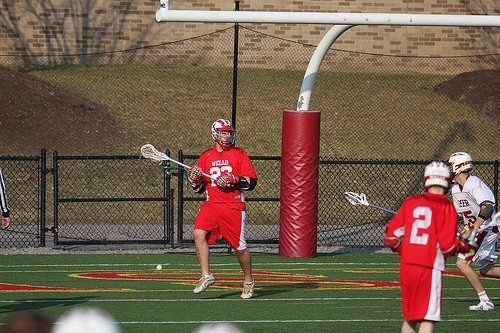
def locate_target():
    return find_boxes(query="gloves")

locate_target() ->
[187,164,203,189]
[215,172,240,190]
[458,223,479,241]
[455,240,470,253]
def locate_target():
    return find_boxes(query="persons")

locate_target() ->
[2,312,51,333]
[0,167,10,230]
[51,305,122,333]
[384,162,469,333]
[188,119,258,299]
[194,322,242,333]
[447,152,500,311]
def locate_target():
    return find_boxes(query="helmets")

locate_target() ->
[446,151,474,183]
[210,118,235,141]
[424,161,451,189]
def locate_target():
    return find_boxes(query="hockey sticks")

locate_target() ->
[140,144,235,188]
[462,212,500,239]
[343,191,477,242]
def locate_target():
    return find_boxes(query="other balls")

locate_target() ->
[157,265,162,270]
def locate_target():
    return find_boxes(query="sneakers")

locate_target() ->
[192,274,215,294]
[468,300,495,312]
[240,277,255,298]
